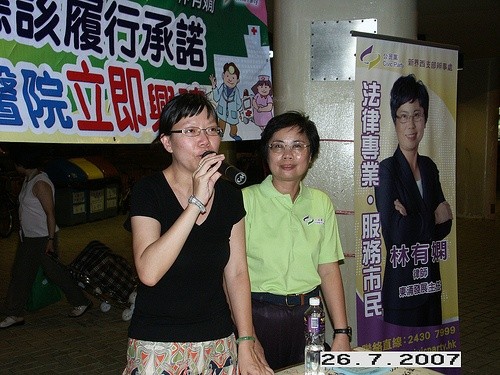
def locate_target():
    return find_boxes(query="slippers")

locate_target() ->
[68,301,93,318]
[0,316,26,329]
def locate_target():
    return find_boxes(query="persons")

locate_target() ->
[122,92,272,375]
[0,146,94,329]
[223,111,350,375]
[375,74,454,326]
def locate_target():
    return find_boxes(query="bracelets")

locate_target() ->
[188,195,206,213]
[48,238,55,241]
[236,336,255,344]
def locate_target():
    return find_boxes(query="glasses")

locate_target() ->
[171,126,221,136]
[266,140,311,153]
[396,113,424,123]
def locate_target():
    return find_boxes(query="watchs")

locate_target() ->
[334,326,352,341]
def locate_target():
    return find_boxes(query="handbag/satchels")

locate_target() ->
[32,264,72,319]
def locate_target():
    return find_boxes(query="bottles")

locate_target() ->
[302,297,326,375]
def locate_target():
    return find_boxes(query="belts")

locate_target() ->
[251,285,320,309]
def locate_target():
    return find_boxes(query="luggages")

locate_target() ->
[66,240,141,321]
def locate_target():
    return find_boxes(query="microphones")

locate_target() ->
[201,151,247,185]
